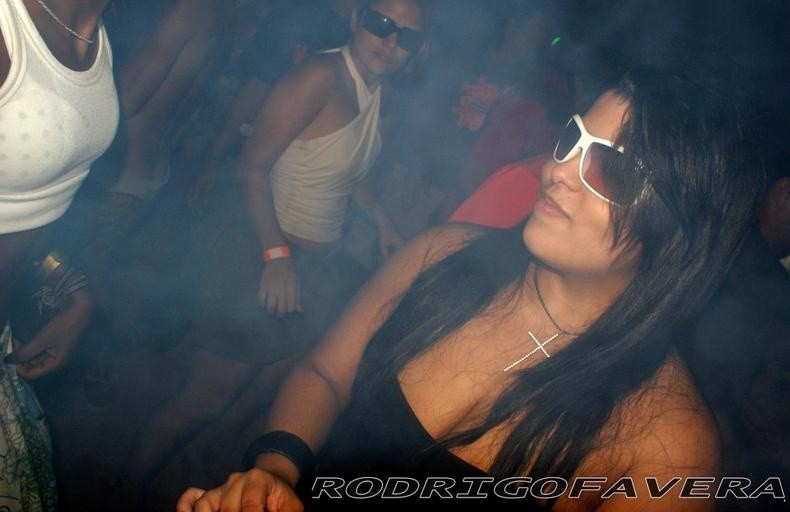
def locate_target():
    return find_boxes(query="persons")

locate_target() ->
[0,0,788,511]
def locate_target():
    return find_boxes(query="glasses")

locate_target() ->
[359,11,423,55]
[551,114,652,209]
